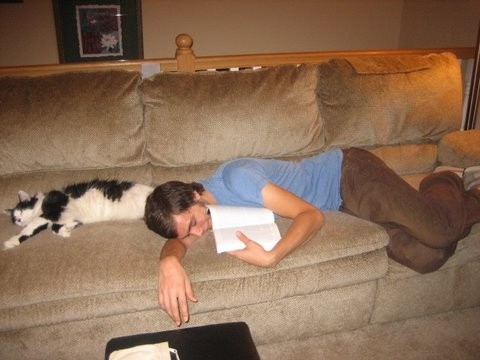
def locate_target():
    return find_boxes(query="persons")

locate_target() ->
[143,146,480,327]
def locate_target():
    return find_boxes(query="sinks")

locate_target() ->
[0,50,480,360]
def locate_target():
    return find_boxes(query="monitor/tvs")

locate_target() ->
[206,203,282,255]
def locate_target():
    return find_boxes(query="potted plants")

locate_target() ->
[52,0,144,64]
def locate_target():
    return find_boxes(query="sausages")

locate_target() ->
[3,178,154,250]
